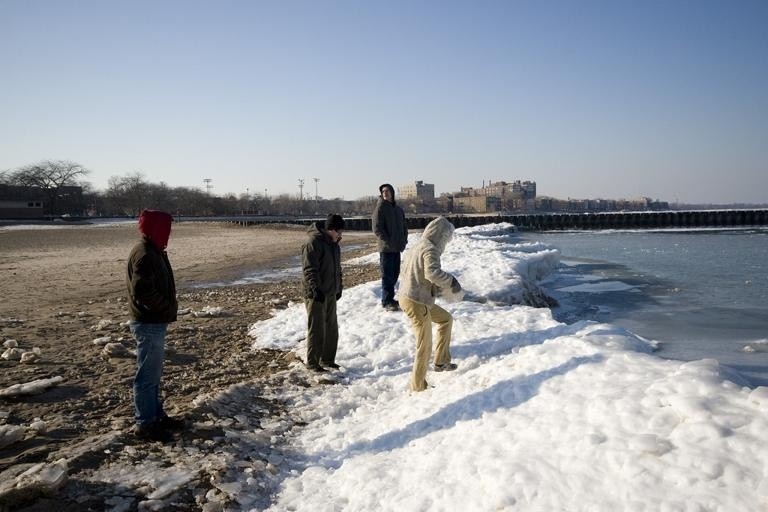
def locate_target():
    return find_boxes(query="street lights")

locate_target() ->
[297,178,304,215]
[246,188,249,215]
[265,188,267,216]
[203,178,213,217]
[312,177,320,215]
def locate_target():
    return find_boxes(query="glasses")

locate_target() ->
[334,229,343,234]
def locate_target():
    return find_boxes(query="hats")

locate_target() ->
[324,214,344,230]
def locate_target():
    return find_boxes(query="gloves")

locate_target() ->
[450,275,461,293]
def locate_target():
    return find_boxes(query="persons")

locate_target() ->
[372,183,409,310]
[399,216,462,391]
[301,214,344,373]
[125,208,178,441]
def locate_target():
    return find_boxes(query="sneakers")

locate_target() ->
[392,299,398,307]
[308,366,329,375]
[433,363,456,372]
[384,303,397,311]
[325,364,340,371]
